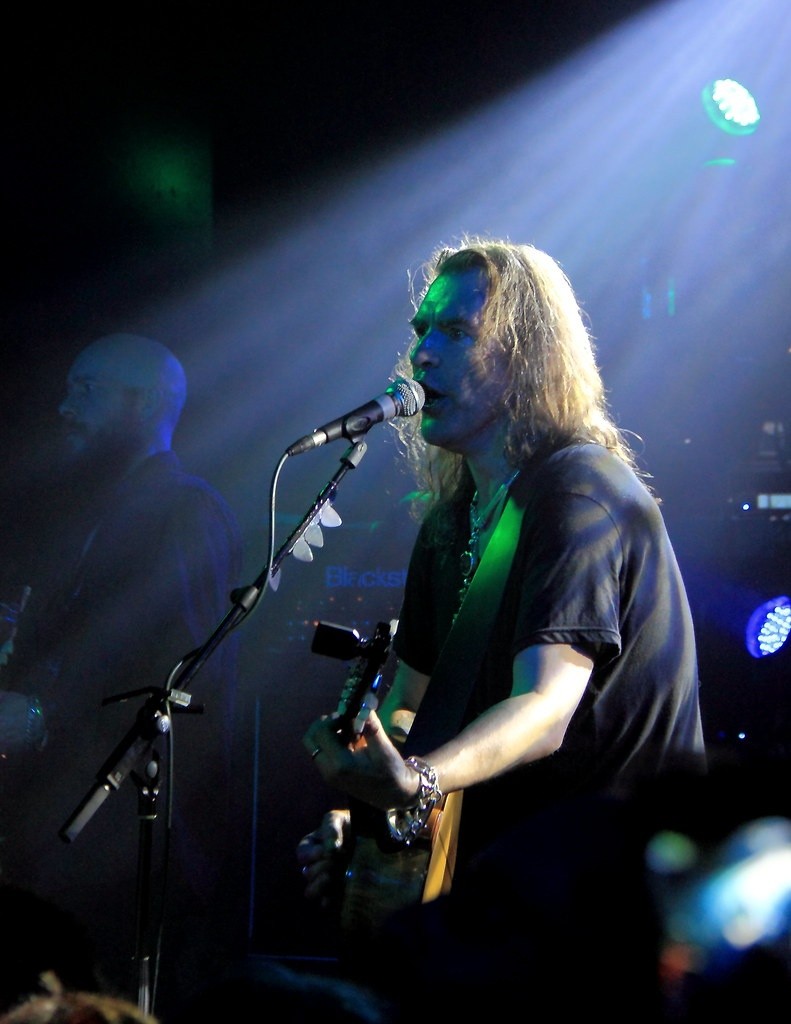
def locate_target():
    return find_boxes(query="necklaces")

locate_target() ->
[450,470,519,625]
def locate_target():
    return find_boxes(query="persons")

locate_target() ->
[1,336,243,1023]
[301,245,709,1023]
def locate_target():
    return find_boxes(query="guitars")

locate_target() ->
[306,616,460,915]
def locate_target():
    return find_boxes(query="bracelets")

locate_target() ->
[385,755,442,845]
[26,695,39,754]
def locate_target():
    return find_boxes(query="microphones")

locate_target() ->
[286,376,427,458]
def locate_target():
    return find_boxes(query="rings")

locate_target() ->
[311,748,319,759]
